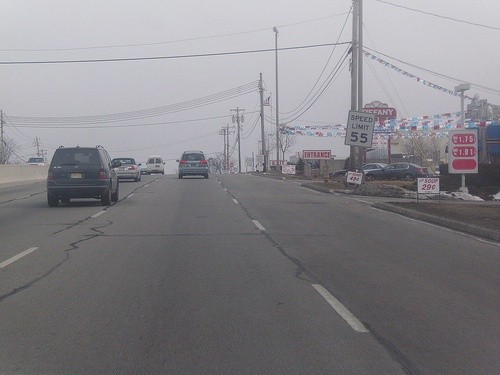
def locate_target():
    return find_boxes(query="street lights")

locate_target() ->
[454,83,470,192]
[272,25,281,174]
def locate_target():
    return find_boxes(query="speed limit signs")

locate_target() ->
[344,110,376,147]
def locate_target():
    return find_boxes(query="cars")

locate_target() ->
[362,162,387,180]
[137,161,147,176]
[110,157,142,181]
[364,161,432,181]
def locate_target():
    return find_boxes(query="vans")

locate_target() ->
[145,156,166,177]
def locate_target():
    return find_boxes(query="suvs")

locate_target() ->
[176,150,209,180]
[25,157,48,166]
[46,144,122,205]
